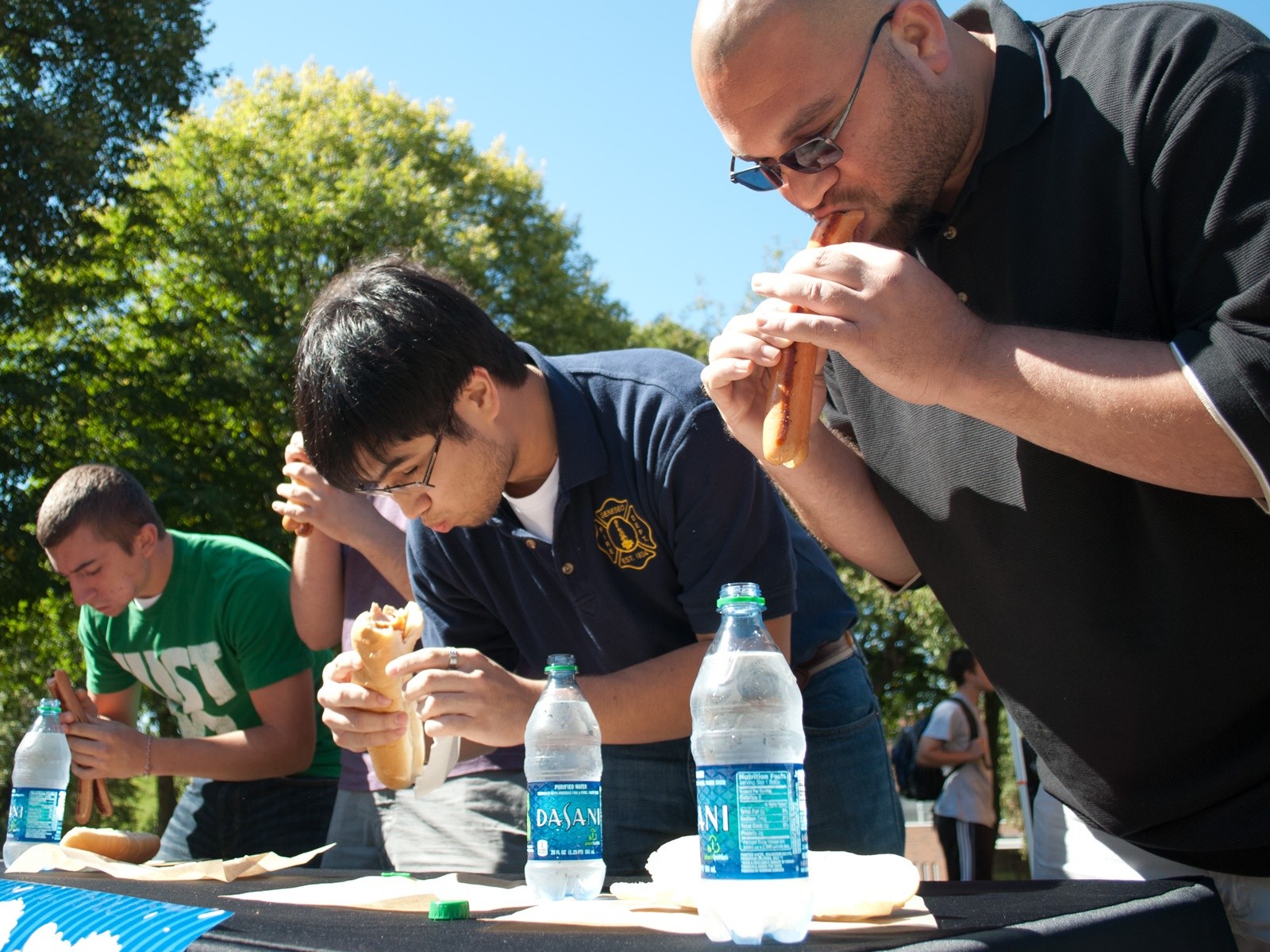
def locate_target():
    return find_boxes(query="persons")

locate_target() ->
[915,646,995,881]
[691,0,1270,952]
[36,464,343,863]
[296,258,904,880]
[270,430,502,868]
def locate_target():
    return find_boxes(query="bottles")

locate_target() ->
[524,653,606,903]
[687,580,813,946]
[3,697,73,872]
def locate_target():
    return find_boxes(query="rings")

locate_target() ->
[446,646,456,669]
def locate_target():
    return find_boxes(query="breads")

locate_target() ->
[60,826,161,864]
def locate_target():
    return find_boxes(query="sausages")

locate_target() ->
[762,209,864,468]
[47,670,113,825]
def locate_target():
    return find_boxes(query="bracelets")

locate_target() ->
[143,734,152,777]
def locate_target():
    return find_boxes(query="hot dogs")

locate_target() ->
[349,600,424,787]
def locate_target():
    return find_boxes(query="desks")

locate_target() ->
[0,860,1240,951]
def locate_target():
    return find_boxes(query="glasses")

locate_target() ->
[731,11,897,191]
[354,400,454,493]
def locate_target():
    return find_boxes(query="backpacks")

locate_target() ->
[892,696,979,800]
